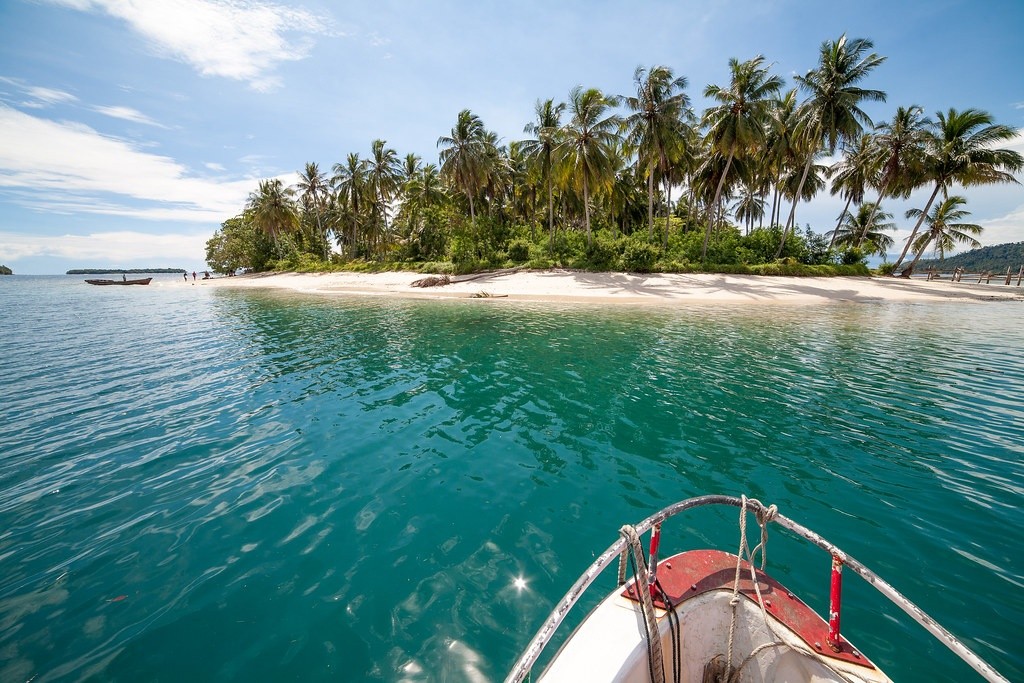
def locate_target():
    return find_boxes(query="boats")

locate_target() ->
[84,277,152,285]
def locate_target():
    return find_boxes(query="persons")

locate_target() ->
[192,271,196,280]
[205,271,209,277]
[184,272,187,282]
[123,273,126,281]
